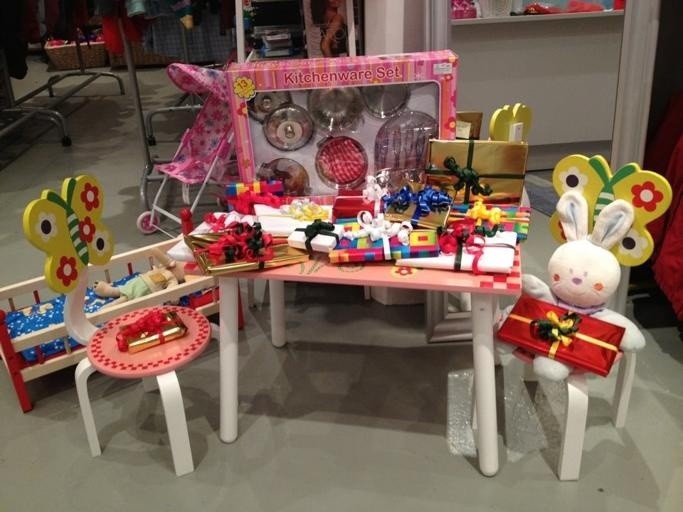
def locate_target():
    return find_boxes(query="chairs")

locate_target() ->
[21,175,220,476]
[487,154,673,481]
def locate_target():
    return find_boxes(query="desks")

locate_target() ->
[167,211,522,477]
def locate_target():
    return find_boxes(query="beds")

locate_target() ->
[0,236,246,414]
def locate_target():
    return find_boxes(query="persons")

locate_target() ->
[310,1,348,58]
